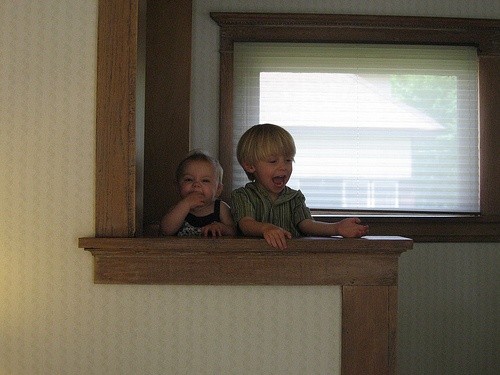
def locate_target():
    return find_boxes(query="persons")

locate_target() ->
[230,124,369,252]
[158,150,240,240]
